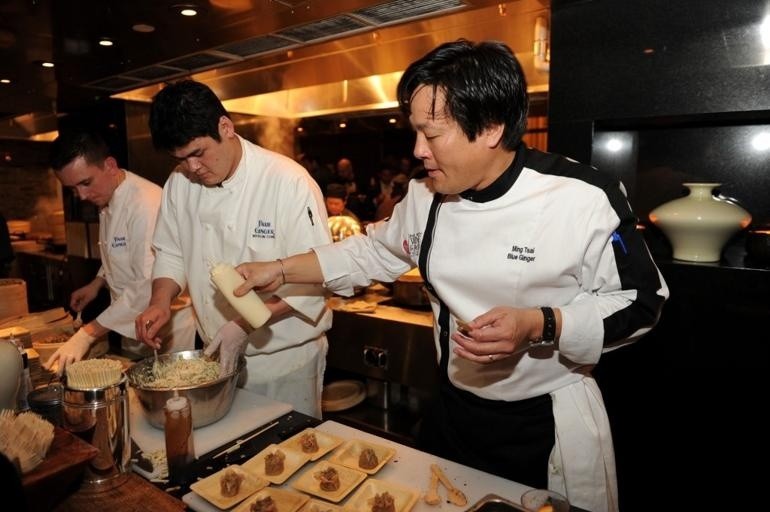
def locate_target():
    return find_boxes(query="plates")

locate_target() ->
[188,428,421,512]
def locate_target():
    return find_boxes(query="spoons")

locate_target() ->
[424,462,469,508]
[146,317,164,380]
[73,304,84,329]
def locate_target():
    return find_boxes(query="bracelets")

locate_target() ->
[276,258,286,285]
[531,306,557,351]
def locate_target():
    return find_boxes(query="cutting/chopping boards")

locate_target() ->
[181,418,394,512]
[104,352,294,481]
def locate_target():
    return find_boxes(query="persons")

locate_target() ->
[133,77,339,423]
[319,154,430,243]
[42,125,199,377]
[231,35,680,512]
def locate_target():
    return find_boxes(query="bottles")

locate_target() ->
[164,387,196,468]
[203,253,273,330]
[16,346,34,412]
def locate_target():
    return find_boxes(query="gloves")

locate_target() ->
[45,328,97,377]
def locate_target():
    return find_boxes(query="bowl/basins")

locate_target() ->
[27,384,62,412]
[130,347,248,432]
[31,326,110,365]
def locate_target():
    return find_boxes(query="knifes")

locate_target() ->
[129,438,154,474]
